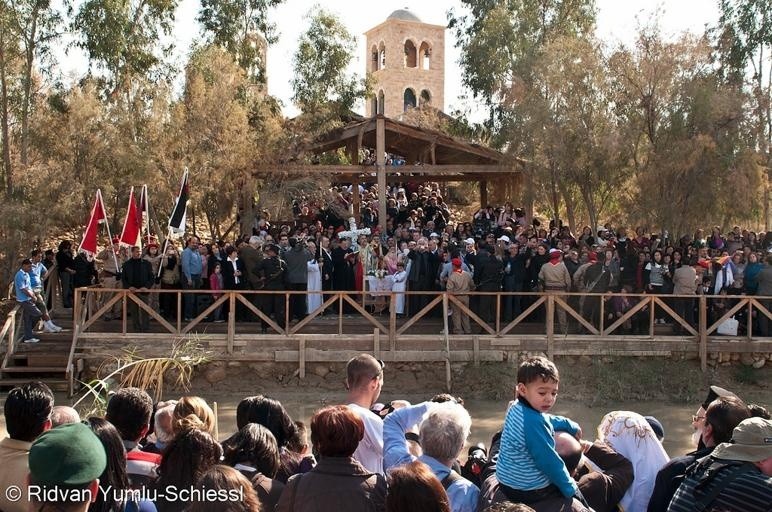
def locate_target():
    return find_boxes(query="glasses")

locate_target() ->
[692,414,705,422]
[371,359,385,380]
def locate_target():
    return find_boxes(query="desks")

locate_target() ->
[362,274,410,317]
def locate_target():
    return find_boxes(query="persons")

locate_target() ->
[80,416,157,512]
[340,353,384,475]
[144,235,154,246]
[121,245,154,333]
[691,385,739,451]
[14,259,43,343]
[28,250,62,333]
[274,404,389,512]
[96,238,122,321]
[186,465,287,512]
[0,380,55,512]
[477,430,635,512]
[598,409,672,512]
[667,417,772,512]
[646,395,751,512]
[209,262,226,323]
[161,245,180,322]
[208,243,227,280]
[140,426,224,511]
[56,239,76,308]
[226,246,242,322]
[216,240,226,258]
[172,395,216,439]
[42,249,54,313]
[143,244,162,321]
[383,401,481,512]
[25,422,107,512]
[291,419,310,454]
[180,236,201,323]
[235,395,294,449]
[218,422,288,512]
[105,387,162,498]
[495,356,593,512]
[236,146,772,338]
[200,245,212,319]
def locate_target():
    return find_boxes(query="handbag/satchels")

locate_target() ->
[717,316,739,336]
[162,264,178,284]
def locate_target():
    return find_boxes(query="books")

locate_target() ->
[344,250,360,260]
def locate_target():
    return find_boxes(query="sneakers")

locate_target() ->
[44,326,55,332]
[50,324,62,331]
[24,338,40,342]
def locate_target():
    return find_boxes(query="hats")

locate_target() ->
[702,385,745,411]
[497,235,509,243]
[589,252,596,259]
[463,237,475,244]
[430,233,437,237]
[552,252,559,258]
[550,248,561,253]
[645,415,664,440]
[712,416,772,462]
[452,258,462,266]
[597,226,608,234]
[113,238,119,244]
[146,239,158,248]
[709,239,724,251]
[28,423,107,491]
[270,243,281,254]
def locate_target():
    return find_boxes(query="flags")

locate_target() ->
[78,191,105,256]
[119,186,142,248]
[168,168,191,236]
